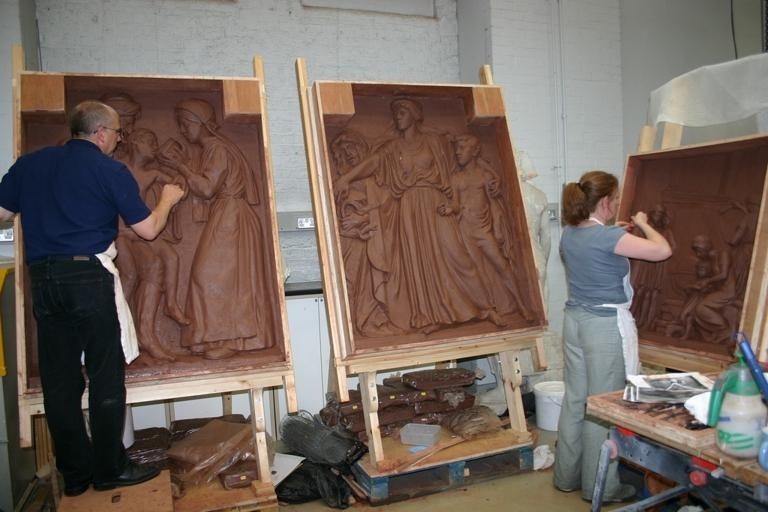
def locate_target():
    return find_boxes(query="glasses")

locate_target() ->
[103,127,123,134]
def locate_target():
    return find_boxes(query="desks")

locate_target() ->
[585,371,768,512]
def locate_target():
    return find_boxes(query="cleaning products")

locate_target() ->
[708,350,768,459]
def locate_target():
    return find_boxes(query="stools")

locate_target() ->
[59,470,173,512]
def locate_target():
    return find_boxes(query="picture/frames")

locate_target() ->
[600,132,768,364]
[16,70,293,396]
[311,80,548,361]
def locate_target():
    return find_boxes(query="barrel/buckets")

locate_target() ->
[534,380,565,432]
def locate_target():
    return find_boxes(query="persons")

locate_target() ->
[725,191,761,295]
[691,233,737,347]
[551,169,674,503]
[118,127,190,328]
[629,203,677,328]
[155,97,277,360]
[331,131,390,336]
[436,123,535,324]
[330,97,507,338]
[678,261,713,323]
[0,99,187,498]
[95,90,179,363]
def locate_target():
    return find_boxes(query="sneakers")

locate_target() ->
[581,483,636,504]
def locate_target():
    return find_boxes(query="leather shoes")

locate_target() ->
[93,459,161,491]
[63,473,91,497]
